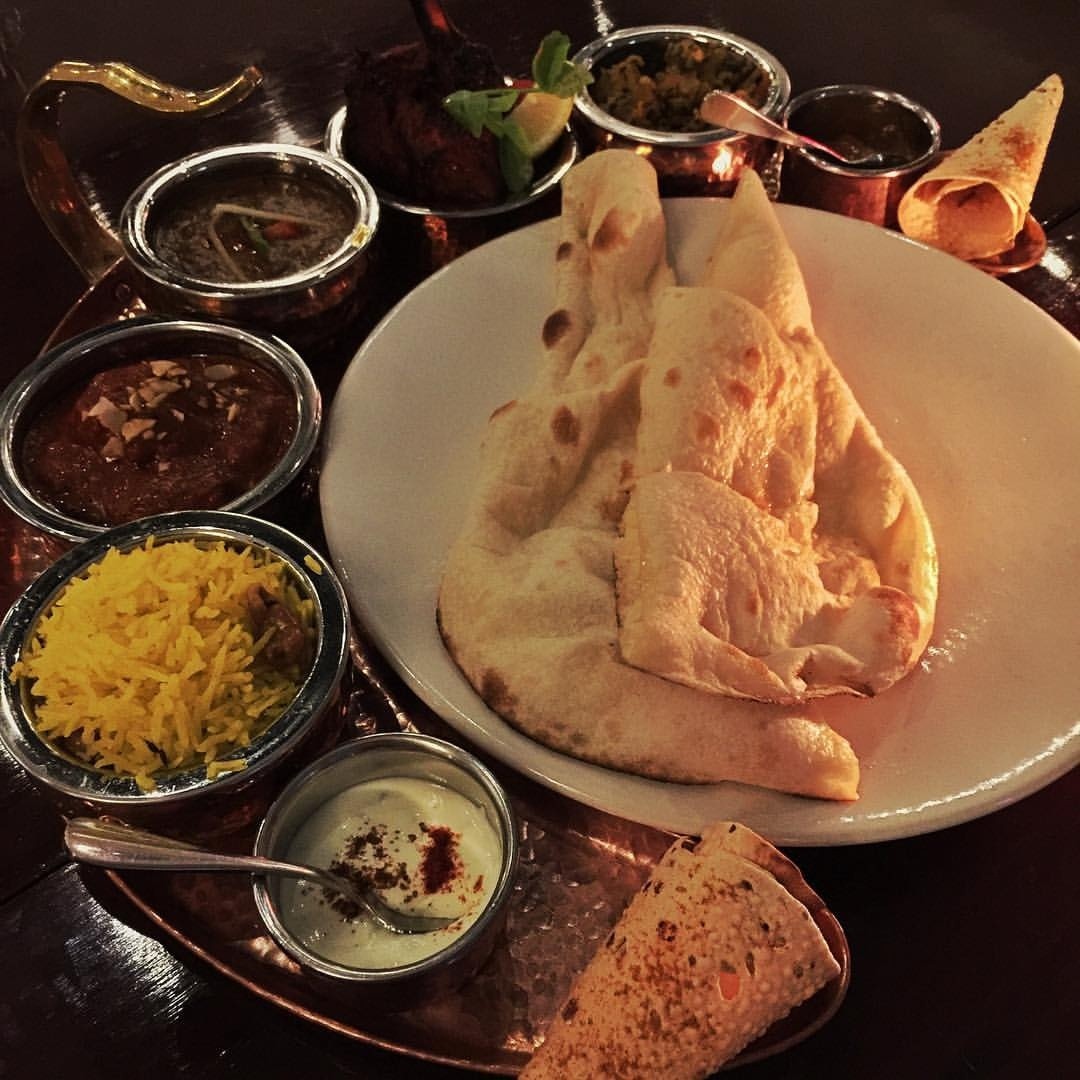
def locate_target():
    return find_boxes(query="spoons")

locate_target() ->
[63,816,464,933]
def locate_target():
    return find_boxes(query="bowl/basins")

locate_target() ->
[0,307,324,543]
[568,26,793,201]
[120,143,383,329]
[1,511,353,814]
[249,732,521,1018]
[324,75,576,222]
[780,84,942,230]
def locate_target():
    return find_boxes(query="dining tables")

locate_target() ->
[0,0,1080,1080]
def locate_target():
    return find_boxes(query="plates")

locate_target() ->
[319,193,1080,849]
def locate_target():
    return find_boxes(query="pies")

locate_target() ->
[431,143,952,813]
